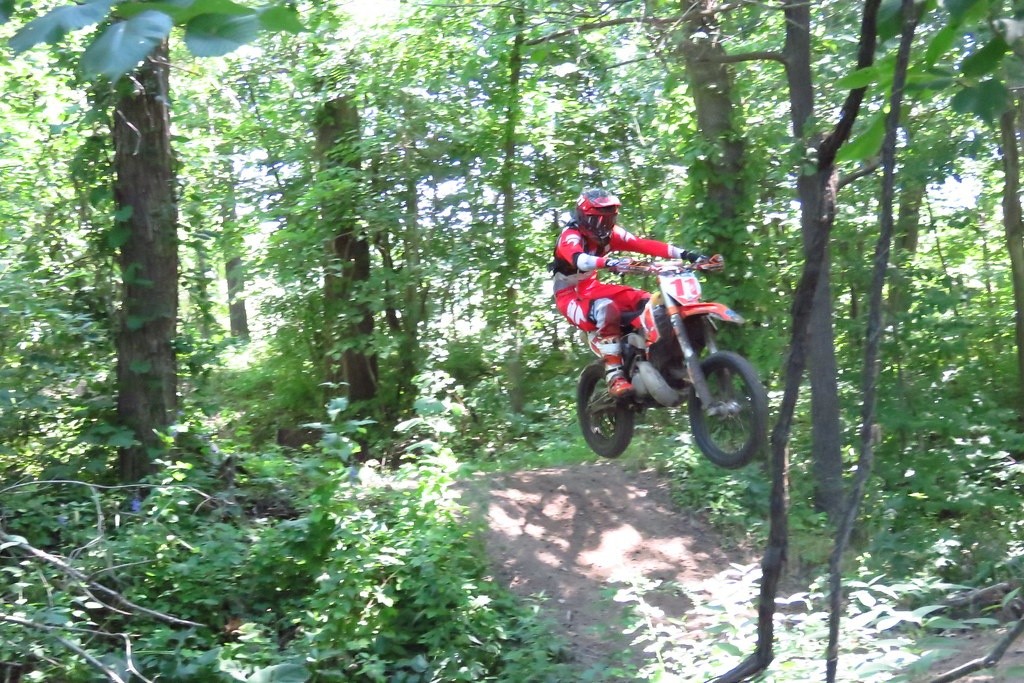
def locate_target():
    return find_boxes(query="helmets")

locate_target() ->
[577,188,621,242]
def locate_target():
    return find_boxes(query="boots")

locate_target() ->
[596,339,636,399]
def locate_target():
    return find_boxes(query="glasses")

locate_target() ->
[577,204,618,230]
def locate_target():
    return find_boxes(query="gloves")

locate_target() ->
[607,258,627,278]
[687,251,709,265]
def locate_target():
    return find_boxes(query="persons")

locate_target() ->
[552,188,711,400]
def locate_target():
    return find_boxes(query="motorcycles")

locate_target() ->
[575,256,770,470]
